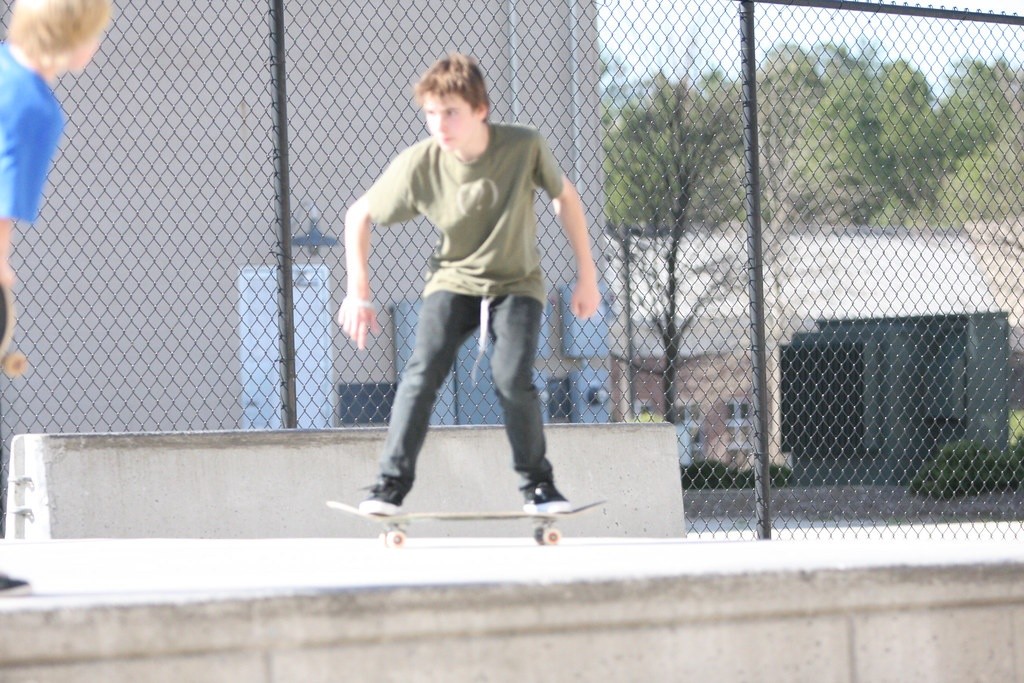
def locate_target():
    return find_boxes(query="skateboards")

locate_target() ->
[0,286,28,380]
[327,500,608,546]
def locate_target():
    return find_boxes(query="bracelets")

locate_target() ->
[343,296,377,309]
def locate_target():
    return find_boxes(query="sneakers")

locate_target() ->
[359,487,402,516]
[524,487,570,514]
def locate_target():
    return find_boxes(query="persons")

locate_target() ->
[334,49,601,519]
[0,0,111,598]
